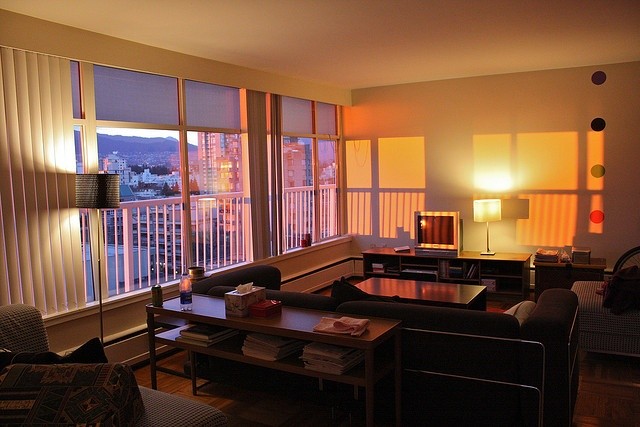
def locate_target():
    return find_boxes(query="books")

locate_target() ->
[466,263,478,278]
[393,245,411,253]
[534,247,559,263]
[387,263,399,272]
[438,259,449,279]
[241,332,304,362]
[371,262,386,274]
[300,341,366,376]
[175,323,240,348]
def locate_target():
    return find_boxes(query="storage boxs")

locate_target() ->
[249,298,282,321]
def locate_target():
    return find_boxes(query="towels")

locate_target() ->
[312,316,370,338]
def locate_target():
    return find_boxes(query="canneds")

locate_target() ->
[151,285,163,308]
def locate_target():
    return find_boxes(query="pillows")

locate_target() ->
[10,349,32,364]
[0,361,145,426]
[502,299,537,326]
[30,350,61,365]
[330,279,400,302]
[63,336,109,364]
[340,275,367,294]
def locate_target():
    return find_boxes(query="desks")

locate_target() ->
[145,293,403,425]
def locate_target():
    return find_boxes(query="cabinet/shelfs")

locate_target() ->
[360,246,532,301]
[532,258,607,302]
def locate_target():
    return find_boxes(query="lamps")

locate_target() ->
[472,198,502,256]
[74,173,120,212]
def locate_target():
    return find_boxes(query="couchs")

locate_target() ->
[571,280,639,357]
[0,303,231,427]
[183,264,581,427]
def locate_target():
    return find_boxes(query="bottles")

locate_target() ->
[179,272,193,311]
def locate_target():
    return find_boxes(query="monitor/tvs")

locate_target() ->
[414,213,459,249]
